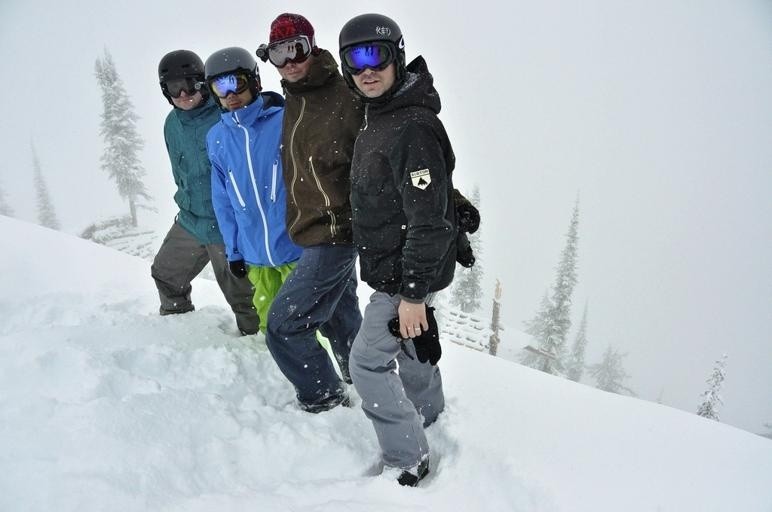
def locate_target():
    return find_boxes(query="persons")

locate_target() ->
[204,46,327,351]
[338,12,460,486]
[149,49,262,334]
[256,12,365,415]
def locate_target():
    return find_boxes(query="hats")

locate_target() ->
[268,12,321,57]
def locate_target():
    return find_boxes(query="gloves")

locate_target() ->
[387,300,443,366]
[227,259,248,280]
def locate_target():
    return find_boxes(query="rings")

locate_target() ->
[414,327,421,331]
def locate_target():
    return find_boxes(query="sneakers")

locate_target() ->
[294,378,355,413]
[379,452,432,487]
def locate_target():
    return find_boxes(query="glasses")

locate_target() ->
[206,66,257,99]
[159,74,205,99]
[267,36,315,68]
[340,41,399,75]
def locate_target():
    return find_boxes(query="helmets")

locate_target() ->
[202,45,263,109]
[154,46,211,104]
[336,13,406,91]
